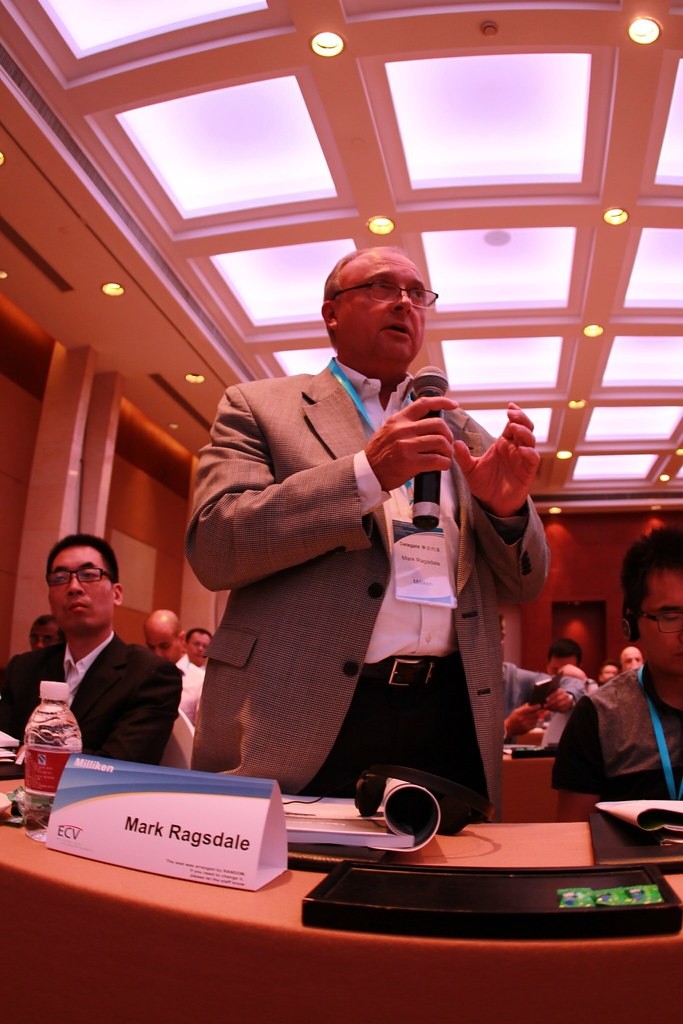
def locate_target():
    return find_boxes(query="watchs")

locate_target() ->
[586,679,595,686]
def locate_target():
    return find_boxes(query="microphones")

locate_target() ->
[411,366,449,531]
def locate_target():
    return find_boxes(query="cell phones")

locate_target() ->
[529,670,564,706]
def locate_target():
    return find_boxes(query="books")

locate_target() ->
[283,779,441,854]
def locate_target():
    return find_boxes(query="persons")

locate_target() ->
[500,622,586,745]
[552,529,683,801]
[187,628,213,668]
[546,641,598,694]
[598,663,618,685]
[29,615,66,650]
[144,610,205,725]
[0,533,182,765]
[183,248,550,813]
[620,647,644,673]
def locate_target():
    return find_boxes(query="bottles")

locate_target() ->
[25,681,82,843]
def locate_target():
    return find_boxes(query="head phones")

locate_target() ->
[621,614,640,644]
[353,764,496,836]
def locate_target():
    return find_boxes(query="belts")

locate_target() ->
[364,652,464,687]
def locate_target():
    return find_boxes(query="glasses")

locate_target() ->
[628,605,683,633]
[331,281,440,308]
[30,633,63,646]
[45,567,115,586]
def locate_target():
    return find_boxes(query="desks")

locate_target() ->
[0,779,683,1024]
[487,744,558,823]
